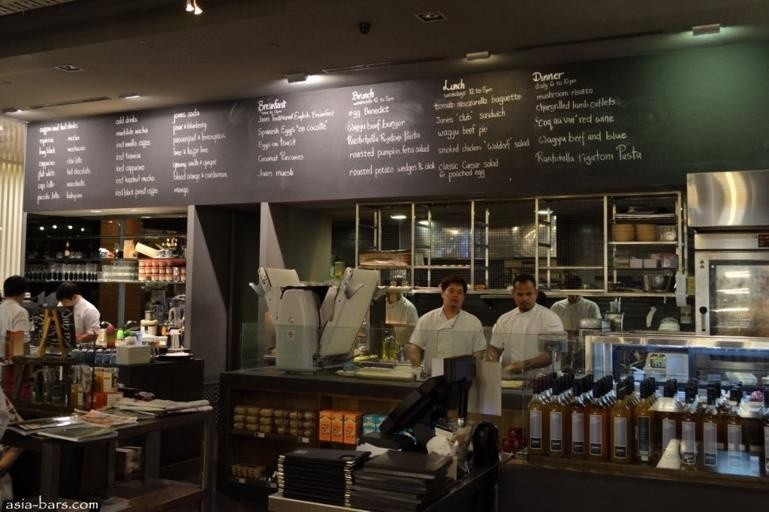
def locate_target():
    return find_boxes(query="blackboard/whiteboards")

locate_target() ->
[23,34,769,211]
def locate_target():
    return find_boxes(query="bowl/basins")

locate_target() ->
[639,272,673,292]
[610,222,657,242]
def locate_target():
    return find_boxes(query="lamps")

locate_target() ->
[183,0,204,19]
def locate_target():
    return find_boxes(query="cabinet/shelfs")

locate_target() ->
[21,168,769,430]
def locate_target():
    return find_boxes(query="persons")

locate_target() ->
[383,291,420,324]
[0,383,35,512]
[485,274,568,374]
[549,274,602,332]
[0,274,34,401]
[409,276,485,366]
[55,280,100,344]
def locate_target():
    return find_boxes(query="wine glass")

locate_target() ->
[23,261,99,282]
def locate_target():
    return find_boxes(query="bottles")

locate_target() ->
[382,329,398,362]
[526,369,769,475]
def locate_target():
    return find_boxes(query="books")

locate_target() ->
[6,391,213,444]
[269,445,461,512]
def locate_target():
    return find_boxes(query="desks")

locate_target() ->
[0,330,768,512]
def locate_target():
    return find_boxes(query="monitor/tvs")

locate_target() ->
[380,375,460,451]
